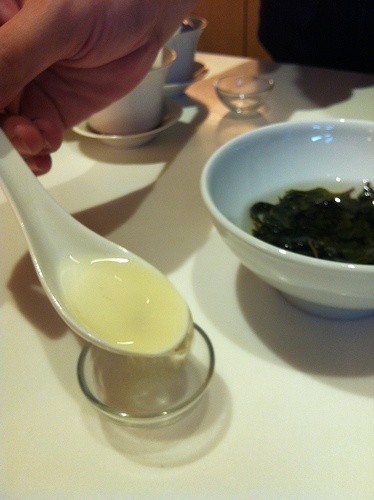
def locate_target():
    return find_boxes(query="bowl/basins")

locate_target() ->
[198,119,374,319]
[77,323,214,427]
[214,74,276,114]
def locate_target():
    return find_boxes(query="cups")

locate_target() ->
[85,47,175,133]
[163,16,208,84]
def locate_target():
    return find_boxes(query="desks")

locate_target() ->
[0,48,374,499]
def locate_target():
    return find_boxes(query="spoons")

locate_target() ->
[0,130,189,356]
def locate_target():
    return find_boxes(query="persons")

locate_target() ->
[0,1,200,176]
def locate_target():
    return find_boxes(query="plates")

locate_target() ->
[162,62,208,97]
[72,97,182,147]
